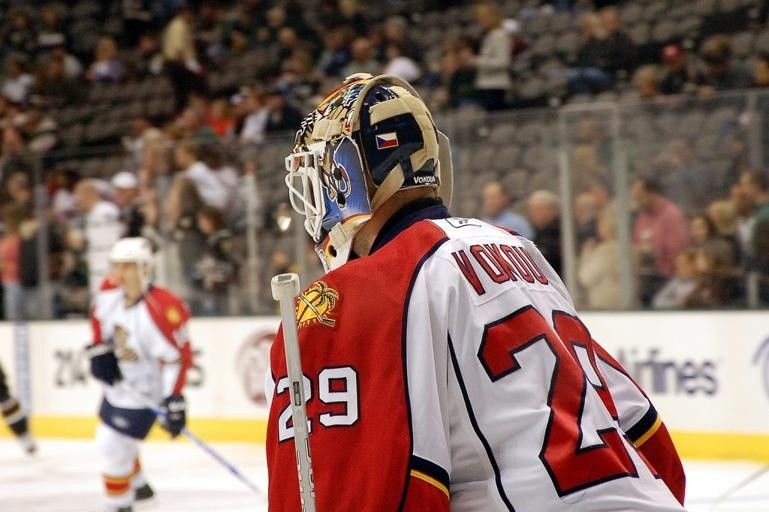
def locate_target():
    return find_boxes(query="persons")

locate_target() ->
[482,68,767,309]
[84,236,193,507]
[2,1,768,161]
[261,70,692,512]
[0,365,37,453]
[2,125,314,321]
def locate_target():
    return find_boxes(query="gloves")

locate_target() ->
[163,395,186,435]
[87,342,123,385]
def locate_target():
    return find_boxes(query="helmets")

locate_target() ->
[286,73,453,272]
[112,236,151,263]
